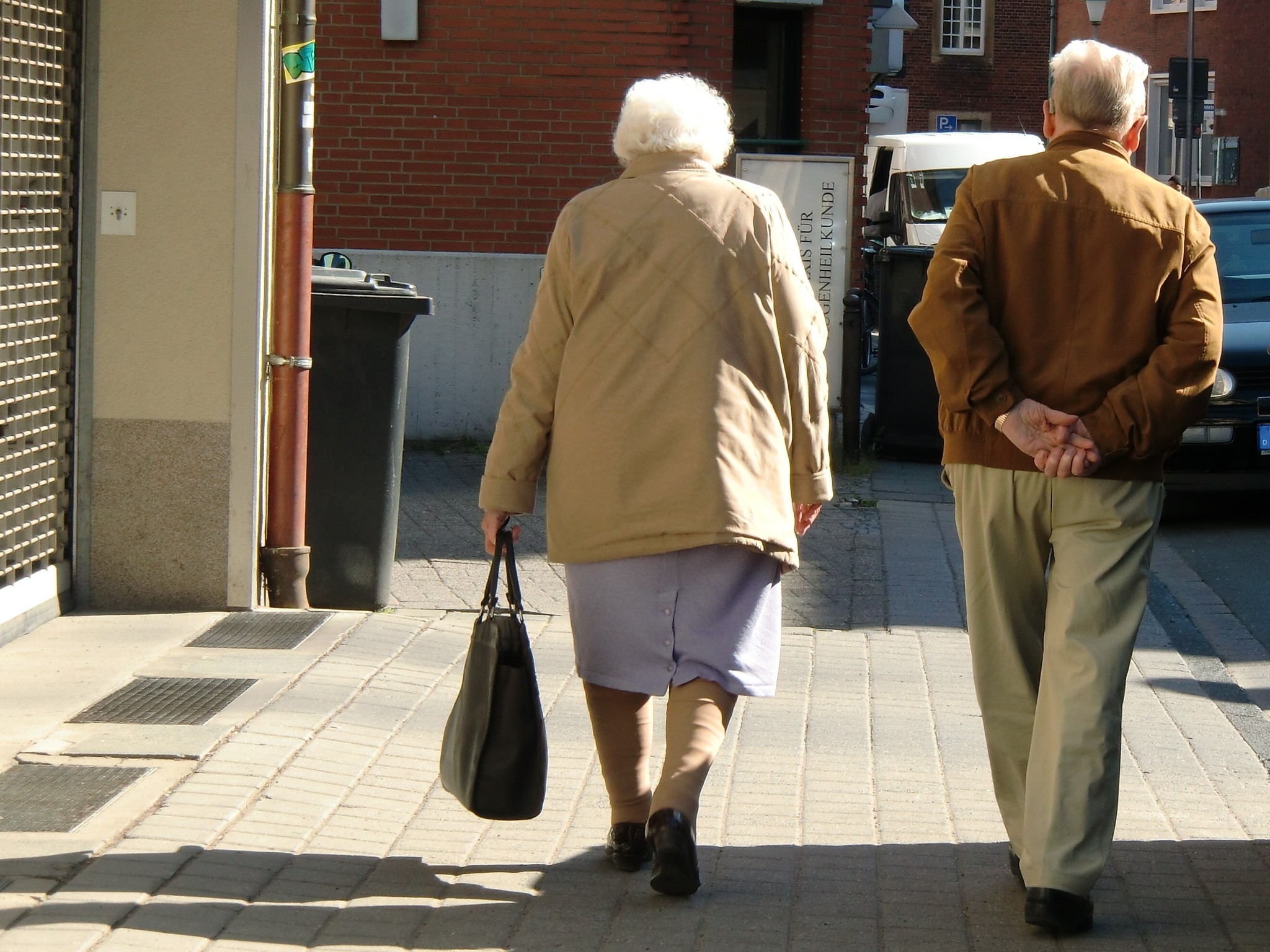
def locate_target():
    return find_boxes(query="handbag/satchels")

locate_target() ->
[437,528,549,824]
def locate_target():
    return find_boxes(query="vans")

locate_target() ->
[864,132,1046,248]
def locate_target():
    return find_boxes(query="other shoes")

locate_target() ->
[1006,840,1027,894]
[1024,886,1095,932]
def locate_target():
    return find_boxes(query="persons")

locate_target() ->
[862,37,1224,934]
[480,75,832,895]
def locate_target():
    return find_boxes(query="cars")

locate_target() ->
[1160,197,1270,494]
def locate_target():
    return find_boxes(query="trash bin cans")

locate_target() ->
[862,245,944,463]
[306,266,433,611]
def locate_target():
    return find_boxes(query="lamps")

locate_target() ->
[867,3,919,31]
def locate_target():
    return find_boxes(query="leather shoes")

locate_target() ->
[604,820,652,873]
[644,808,702,896]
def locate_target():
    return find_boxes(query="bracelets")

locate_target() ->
[994,407,1013,431]
